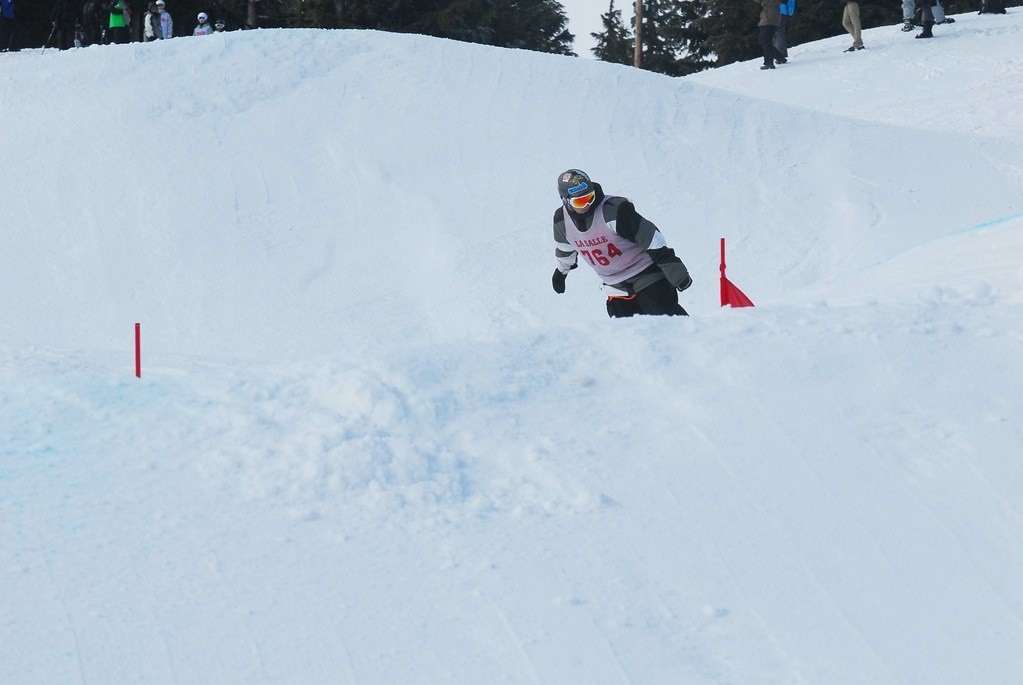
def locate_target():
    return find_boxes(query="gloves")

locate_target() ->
[551,268,567,294]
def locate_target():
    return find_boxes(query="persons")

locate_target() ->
[156,0,173,39]
[193,12,213,35]
[754,0,795,70]
[841,0,865,51]
[900,0,955,31]
[552,168,693,316]
[0,0,132,51]
[212,19,225,33]
[143,2,163,42]
[916,0,936,38]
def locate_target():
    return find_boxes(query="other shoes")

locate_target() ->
[915,33,932,38]
[857,46,865,50]
[843,47,855,52]
[775,59,788,64]
[760,64,775,70]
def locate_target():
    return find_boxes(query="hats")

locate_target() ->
[557,168,593,199]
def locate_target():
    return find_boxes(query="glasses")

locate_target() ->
[199,17,204,20]
[215,24,225,29]
[570,190,596,208]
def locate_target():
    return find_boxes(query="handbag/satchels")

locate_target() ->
[930,0,945,23]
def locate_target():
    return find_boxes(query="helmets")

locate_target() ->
[197,12,208,20]
[155,0,165,5]
[216,19,225,24]
[147,2,158,7]
